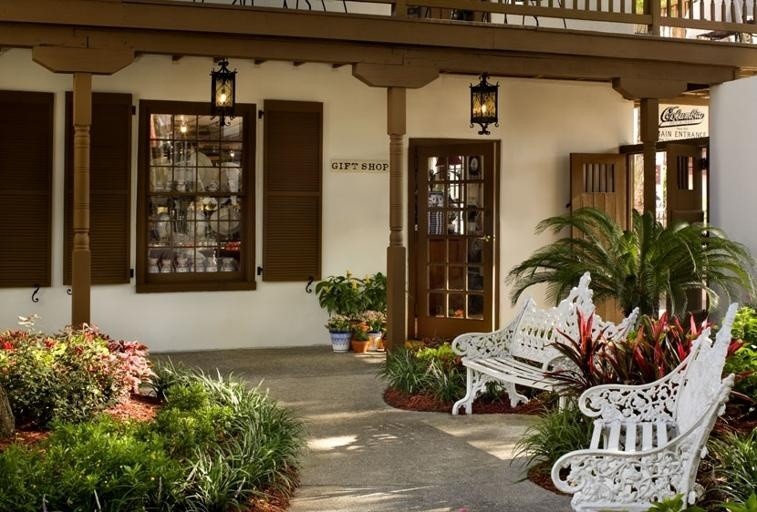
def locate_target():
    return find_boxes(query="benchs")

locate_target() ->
[451,271,639,418]
[551,302,740,511]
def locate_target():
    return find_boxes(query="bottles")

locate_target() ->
[148,257,234,272]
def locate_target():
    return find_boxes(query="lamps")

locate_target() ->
[467,73,500,135]
[209,59,237,127]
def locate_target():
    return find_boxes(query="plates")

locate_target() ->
[149,150,241,242]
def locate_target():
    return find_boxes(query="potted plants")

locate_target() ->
[315,267,388,352]
